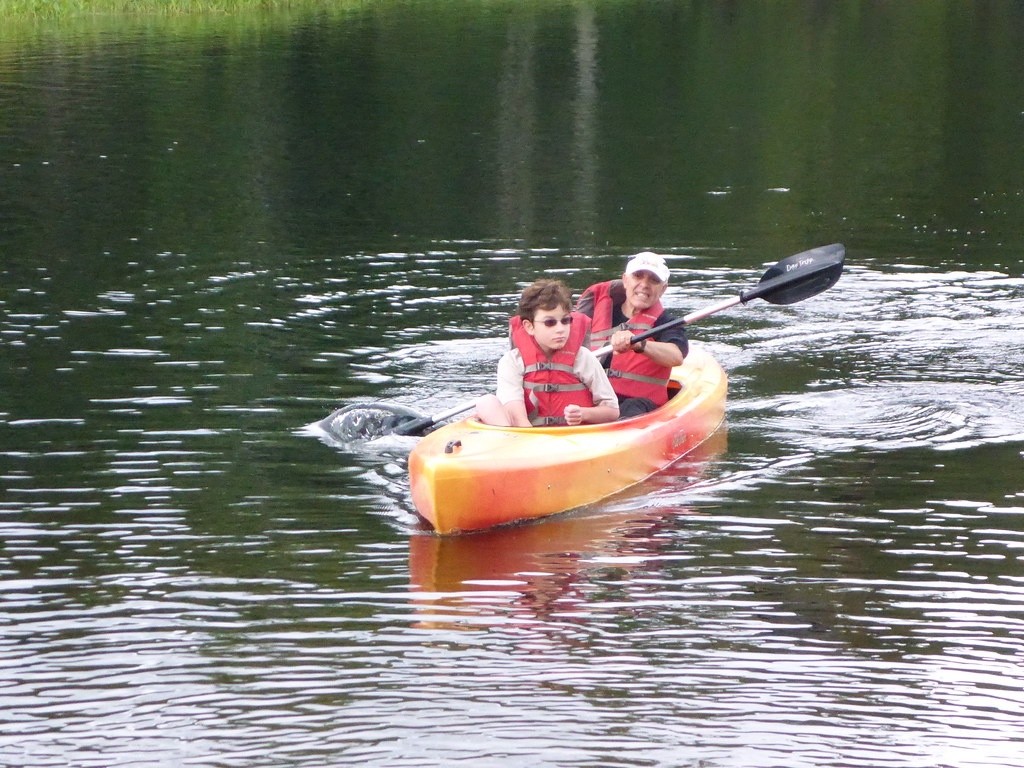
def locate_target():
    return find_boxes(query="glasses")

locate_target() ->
[533,316,573,328]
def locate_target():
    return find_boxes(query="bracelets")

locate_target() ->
[634,339,646,353]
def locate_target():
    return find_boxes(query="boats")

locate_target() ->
[409,339,726,537]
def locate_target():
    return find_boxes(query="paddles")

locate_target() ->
[395,243,845,441]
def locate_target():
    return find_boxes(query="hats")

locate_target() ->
[625,251,670,282]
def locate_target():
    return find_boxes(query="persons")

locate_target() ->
[476,251,689,429]
